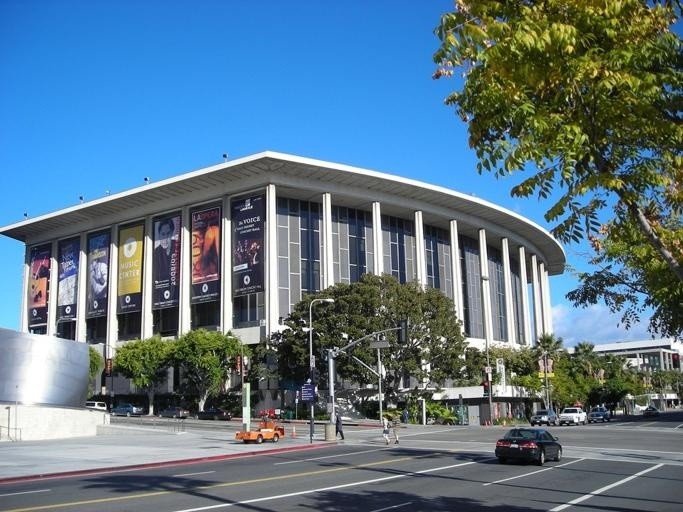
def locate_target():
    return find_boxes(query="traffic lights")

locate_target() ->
[672,353,680,368]
[483,381,488,393]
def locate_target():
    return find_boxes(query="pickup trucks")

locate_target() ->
[559,407,586,426]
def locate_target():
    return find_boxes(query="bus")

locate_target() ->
[86,401,107,410]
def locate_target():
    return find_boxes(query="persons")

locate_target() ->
[153,219,176,283]
[403,407,410,424]
[382,415,391,445]
[335,411,344,440]
[392,411,400,444]
[89,248,108,300]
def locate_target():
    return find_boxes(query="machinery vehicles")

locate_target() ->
[235,419,284,443]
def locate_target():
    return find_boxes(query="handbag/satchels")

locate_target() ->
[388,424,391,428]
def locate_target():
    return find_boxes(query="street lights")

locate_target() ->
[309,297,335,433]
[482,275,495,420]
[226,336,243,388]
[99,343,112,404]
[543,347,563,407]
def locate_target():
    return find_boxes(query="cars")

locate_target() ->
[644,407,658,416]
[158,406,189,418]
[111,403,144,417]
[198,408,231,420]
[531,410,557,426]
[495,426,563,467]
[587,406,610,422]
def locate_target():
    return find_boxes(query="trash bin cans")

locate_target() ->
[286,410,296,419]
